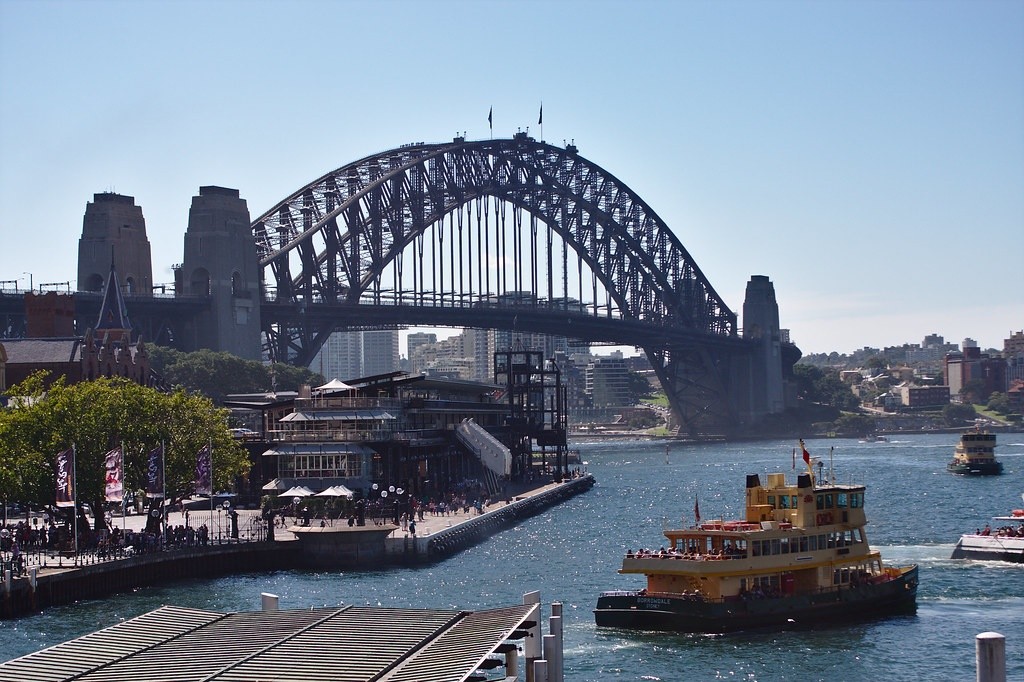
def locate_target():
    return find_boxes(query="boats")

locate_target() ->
[875,436,891,444]
[945,427,1004,476]
[591,438,920,633]
[951,509,1024,563]
[858,433,877,443]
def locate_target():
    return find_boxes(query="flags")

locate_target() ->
[55,448,76,508]
[538,105,542,124]
[695,501,701,523]
[146,445,164,498]
[105,446,123,502]
[488,108,492,129]
[195,444,211,496]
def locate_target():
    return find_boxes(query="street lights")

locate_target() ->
[346,494,354,526]
[371,483,402,524]
[222,501,230,538]
[0,529,10,561]
[151,510,160,547]
[215,504,223,544]
[292,496,301,526]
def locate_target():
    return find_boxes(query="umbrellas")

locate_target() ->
[277,485,355,505]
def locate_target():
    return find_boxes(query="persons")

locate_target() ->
[401,492,485,536]
[321,517,326,527]
[739,584,780,599]
[626,544,746,561]
[637,589,648,595]
[275,516,287,529]
[682,589,704,602]
[526,466,588,485]
[849,571,875,590]
[0,508,208,581]
[976,523,1024,538]
[347,514,356,527]
